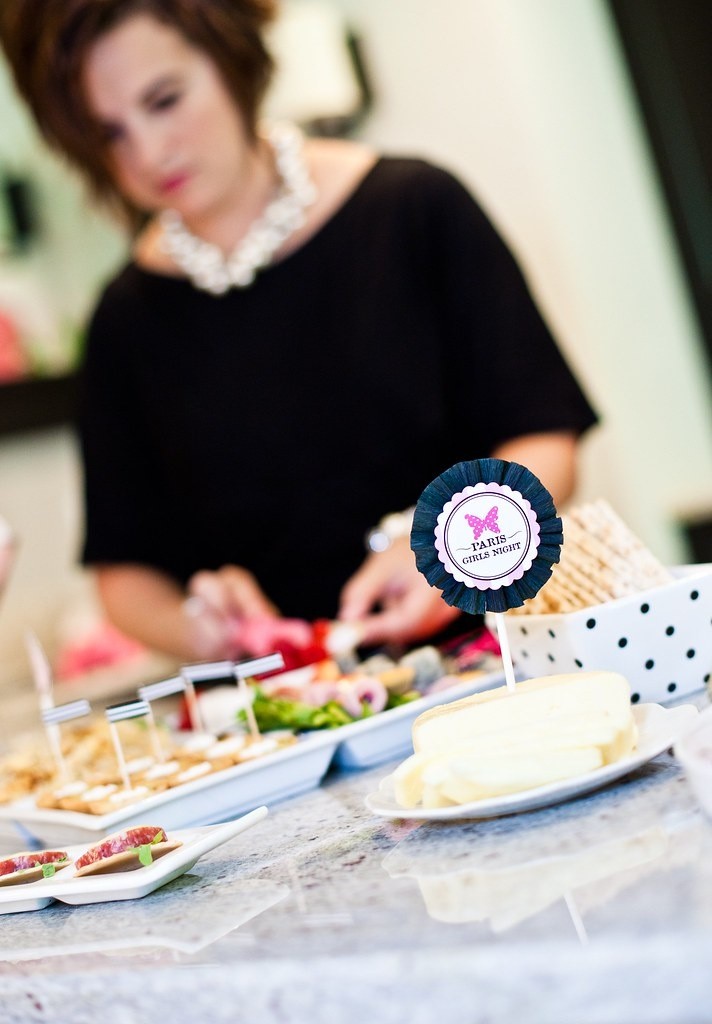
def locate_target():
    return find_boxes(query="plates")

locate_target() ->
[0,810,270,923]
[333,662,509,771]
[363,703,700,820]
[0,727,340,854]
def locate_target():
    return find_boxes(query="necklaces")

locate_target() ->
[154,120,315,296]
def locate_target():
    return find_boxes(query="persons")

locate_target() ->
[1,0,600,663]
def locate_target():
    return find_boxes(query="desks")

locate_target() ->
[0,684,712,1024]
